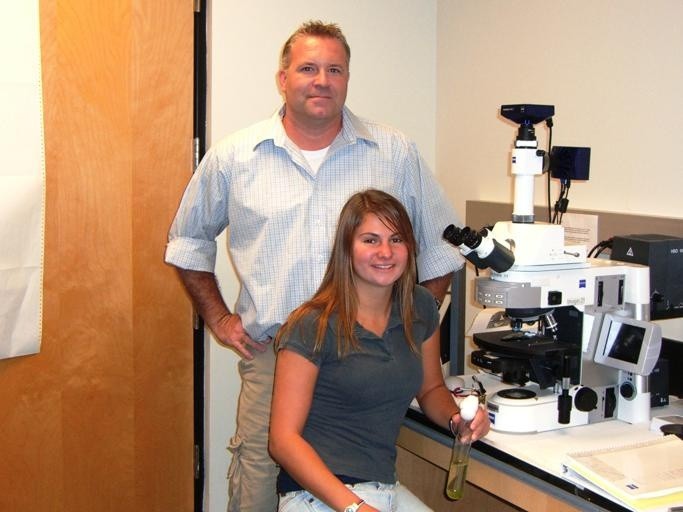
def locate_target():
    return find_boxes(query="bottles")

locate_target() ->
[445,395,479,500]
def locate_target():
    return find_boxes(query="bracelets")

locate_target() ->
[449,417,457,438]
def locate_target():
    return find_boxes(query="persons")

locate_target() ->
[263,186,491,512]
[162,19,468,512]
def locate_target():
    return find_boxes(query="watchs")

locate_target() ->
[343,499,366,512]
